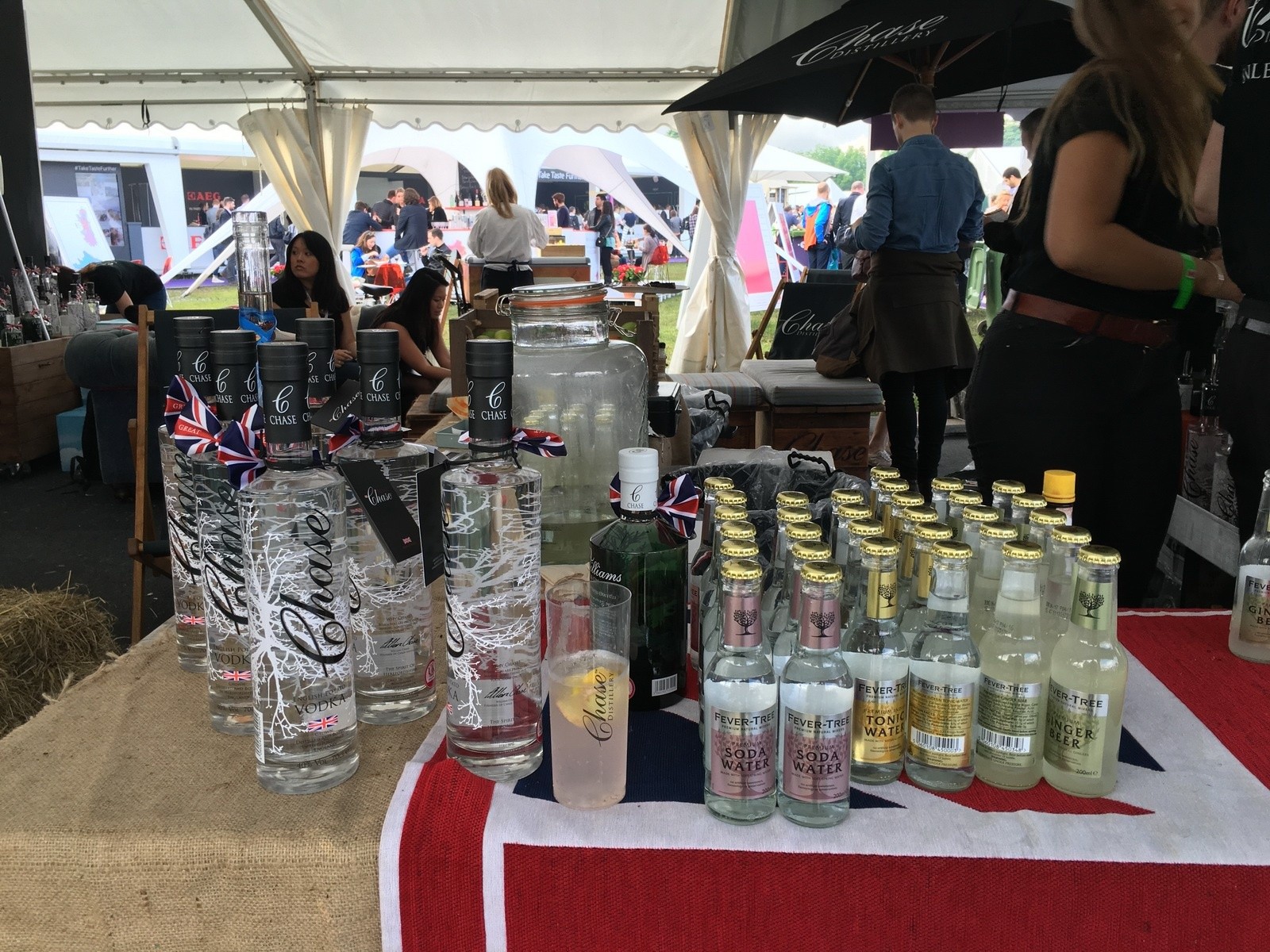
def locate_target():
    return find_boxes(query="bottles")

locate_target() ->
[657,343,667,382]
[370,212,379,231]
[692,463,1128,831]
[0,254,101,349]
[1228,467,1270,666]
[590,446,690,711]
[164,301,538,793]
[231,209,296,344]
[455,188,481,207]
[1178,346,1197,476]
[1182,345,1226,512]
[1209,432,1240,525]
[495,280,650,571]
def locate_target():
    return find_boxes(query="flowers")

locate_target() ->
[269,264,286,279]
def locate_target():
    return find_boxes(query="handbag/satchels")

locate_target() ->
[809,302,863,381]
[595,235,607,248]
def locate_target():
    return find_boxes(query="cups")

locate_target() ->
[545,579,631,810]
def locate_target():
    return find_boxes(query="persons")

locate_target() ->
[853,82,984,501]
[467,168,548,305]
[269,230,359,392]
[55,260,167,333]
[342,187,453,299]
[962,0,1270,615]
[613,199,700,274]
[535,191,614,286]
[197,194,297,285]
[370,268,450,425]
[776,180,870,283]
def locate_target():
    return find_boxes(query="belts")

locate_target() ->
[1003,287,1181,348]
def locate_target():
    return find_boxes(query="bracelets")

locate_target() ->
[1174,253,1196,310]
[1207,261,1225,286]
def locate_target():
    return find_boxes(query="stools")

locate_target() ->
[669,371,768,450]
[401,248,418,271]
[740,359,884,480]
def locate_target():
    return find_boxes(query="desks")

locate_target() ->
[0,411,1270,952]
[623,248,639,268]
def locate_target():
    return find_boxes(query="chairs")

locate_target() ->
[746,268,864,359]
[374,263,405,304]
[648,246,668,280]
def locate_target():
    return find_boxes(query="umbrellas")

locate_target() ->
[749,143,848,183]
[661,0,1095,129]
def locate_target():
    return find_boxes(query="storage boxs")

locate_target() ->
[0,336,82,465]
[56,405,86,472]
